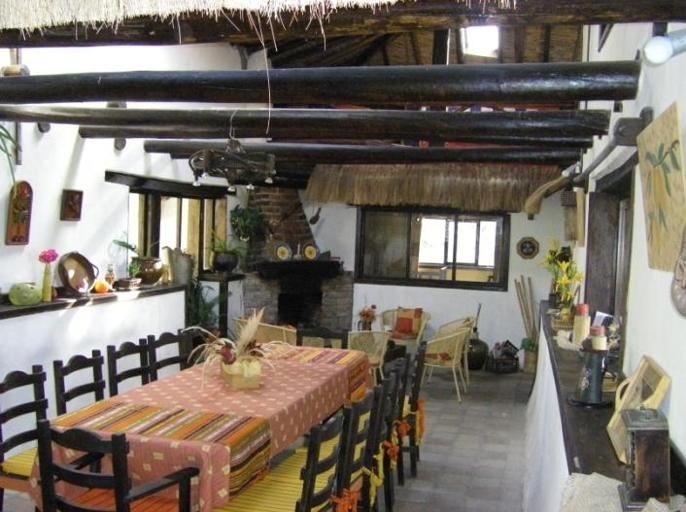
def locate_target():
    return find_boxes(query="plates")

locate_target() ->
[273,241,292,261]
[302,243,319,261]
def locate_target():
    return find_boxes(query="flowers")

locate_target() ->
[552,260,585,307]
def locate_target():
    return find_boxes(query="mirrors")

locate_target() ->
[352,204,511,293]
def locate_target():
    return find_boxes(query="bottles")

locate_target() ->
[588,325,608,367]
[571,302,590,343]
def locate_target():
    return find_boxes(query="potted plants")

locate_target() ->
[207,228,246,273]
[117,238,166,289]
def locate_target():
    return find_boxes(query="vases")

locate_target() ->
[559,303,570,324]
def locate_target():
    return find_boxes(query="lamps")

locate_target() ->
[187,108,280,196]
